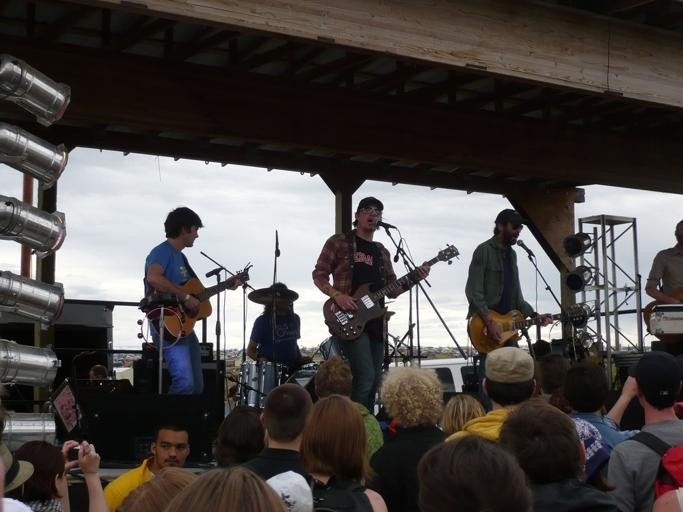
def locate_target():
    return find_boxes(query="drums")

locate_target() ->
[236,361,289,409]
[319,335,351,365]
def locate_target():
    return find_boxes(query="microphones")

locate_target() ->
[392,239,404,264]
[205,267,224,279]
[273,230,281,259]
[372,217,396,231]
[517,238,535,257]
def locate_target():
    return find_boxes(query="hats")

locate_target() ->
[0,443,34,493]
[485,346,534,384]
[495,209,525,224]
[357,197,383,212]
[627,351,681,397]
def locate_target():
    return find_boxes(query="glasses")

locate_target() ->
[356,206,381,215]
[511,223,524,231]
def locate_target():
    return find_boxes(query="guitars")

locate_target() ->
[467,308,584,354]
[323,245,459,342]
[147,263,253,342]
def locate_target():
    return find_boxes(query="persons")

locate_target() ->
[466,210,553,410]
[645,220,683,355]
[247,282,302,371]
[144,207,242,394]
[312,197,429,415]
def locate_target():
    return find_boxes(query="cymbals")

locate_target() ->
[248,288,299,306]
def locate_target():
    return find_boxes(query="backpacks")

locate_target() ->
[630,432,683,498]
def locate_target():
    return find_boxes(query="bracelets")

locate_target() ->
[181,293,190,305]
[332,290,341,298]
[486,319,493,326]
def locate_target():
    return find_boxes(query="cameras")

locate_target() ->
[68,446,84,462]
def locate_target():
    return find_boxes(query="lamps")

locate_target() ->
[560,231,595,353]
[0,53,72,450]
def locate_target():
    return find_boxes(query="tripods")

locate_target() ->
[387,305,428,364]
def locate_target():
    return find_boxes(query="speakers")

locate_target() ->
[102,391,208,462]
[436,367,455,397]
[461,365,476,386]
